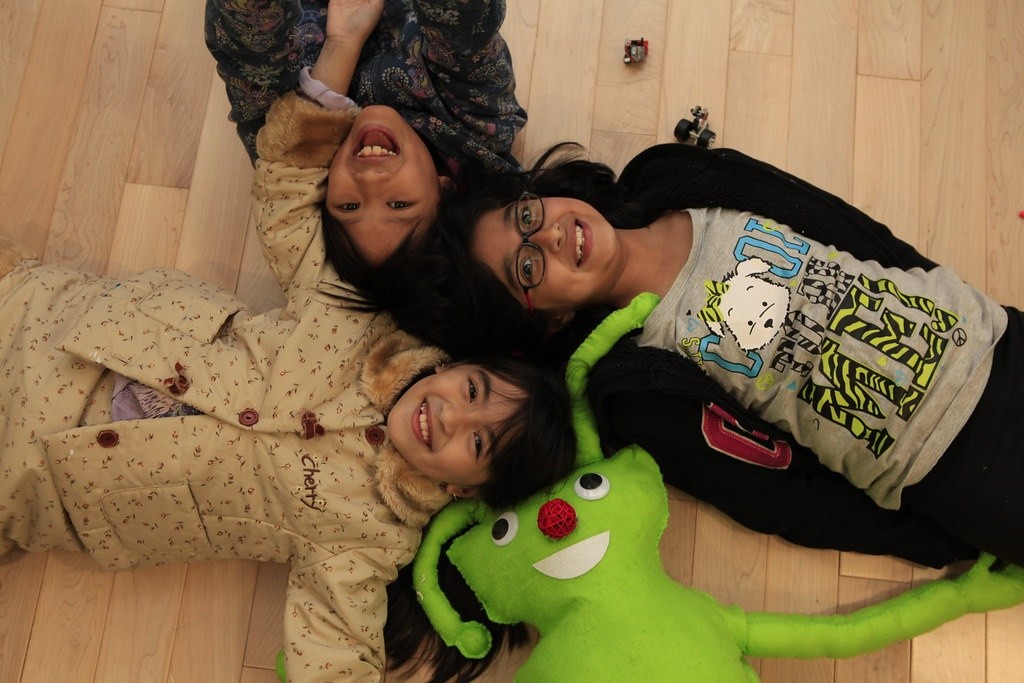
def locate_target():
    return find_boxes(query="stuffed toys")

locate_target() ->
[412,287,1023,683]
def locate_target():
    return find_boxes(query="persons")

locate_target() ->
[1,1,1023,683]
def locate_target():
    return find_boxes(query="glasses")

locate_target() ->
[514,190,546,313]
